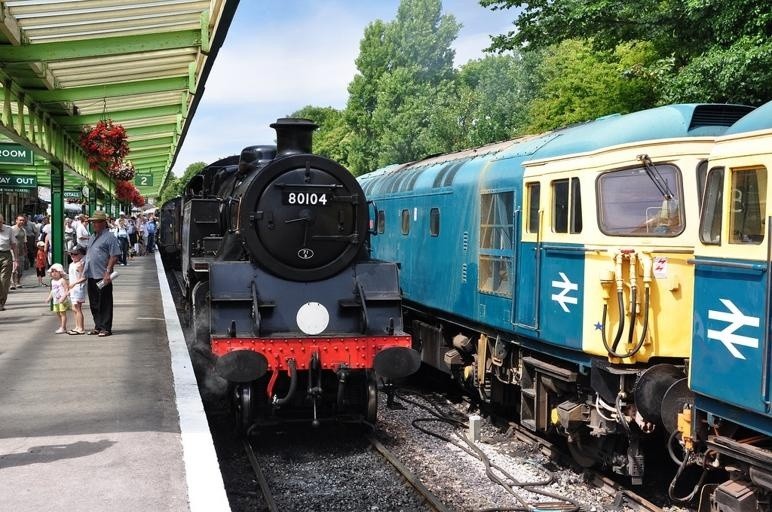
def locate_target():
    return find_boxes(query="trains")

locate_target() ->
[154,118,421,441]
[351,100,771,511]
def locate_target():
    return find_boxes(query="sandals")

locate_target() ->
[88,330,110,336]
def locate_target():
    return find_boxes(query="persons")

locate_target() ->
[0,210,160,337]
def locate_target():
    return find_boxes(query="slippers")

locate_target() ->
[66,330,85,335]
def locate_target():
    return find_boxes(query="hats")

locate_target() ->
[68,246,86,255]
[37,241,45,247]
[88,211,106,220]
[47,264,66,275]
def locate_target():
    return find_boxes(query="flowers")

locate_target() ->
[107,159,136,182]
[114,182,146,208]
[78,96,131,170]
